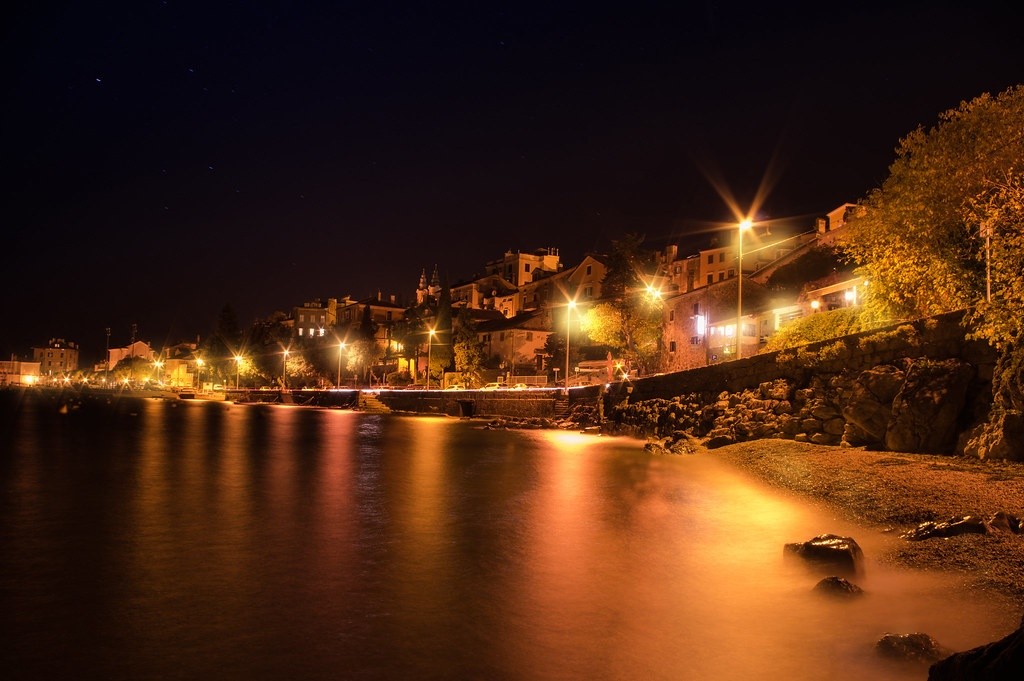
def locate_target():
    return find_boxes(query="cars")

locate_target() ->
[260,386,271,390]
[214,384,223,389]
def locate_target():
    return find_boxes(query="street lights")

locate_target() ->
[337,343,345,389]
[565,300,576,396]
[155,361,163,386]
[196,359,204,392]
[282,351,289,389]
[736,218,754,360]
[236,355,242,390]
[426,330,435,390]
